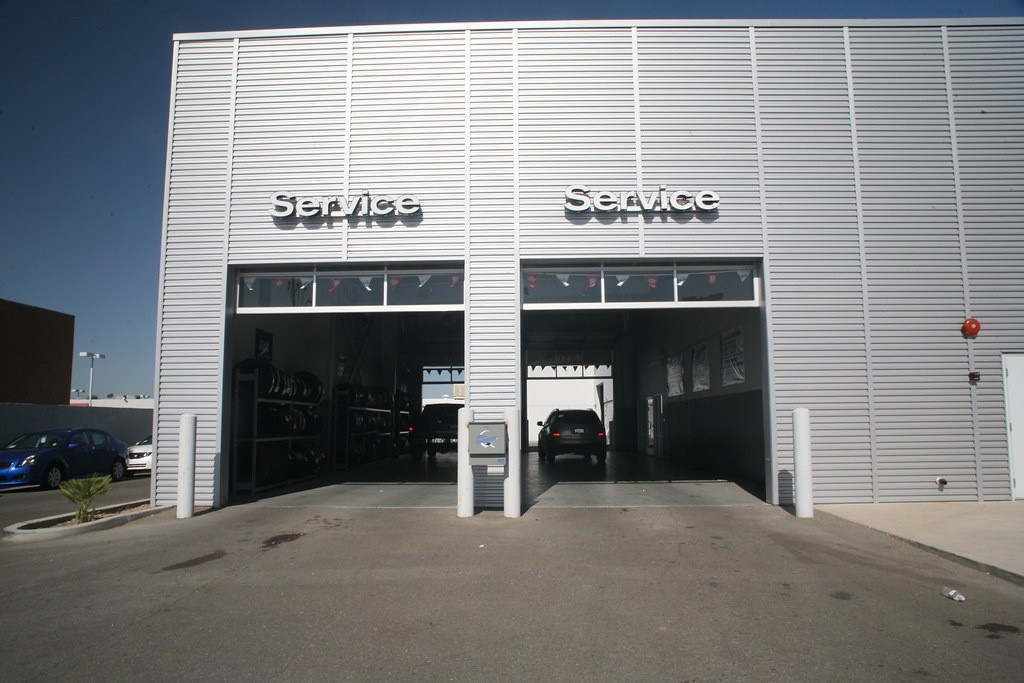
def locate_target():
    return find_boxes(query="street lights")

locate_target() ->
[71,388,86,400]
[79,352,106,407]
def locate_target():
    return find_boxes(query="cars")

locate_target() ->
[0,428,130,489]
[408,404,465,456]
[126,434,152,477]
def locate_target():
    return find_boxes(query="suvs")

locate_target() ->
[537,410,607,462]
